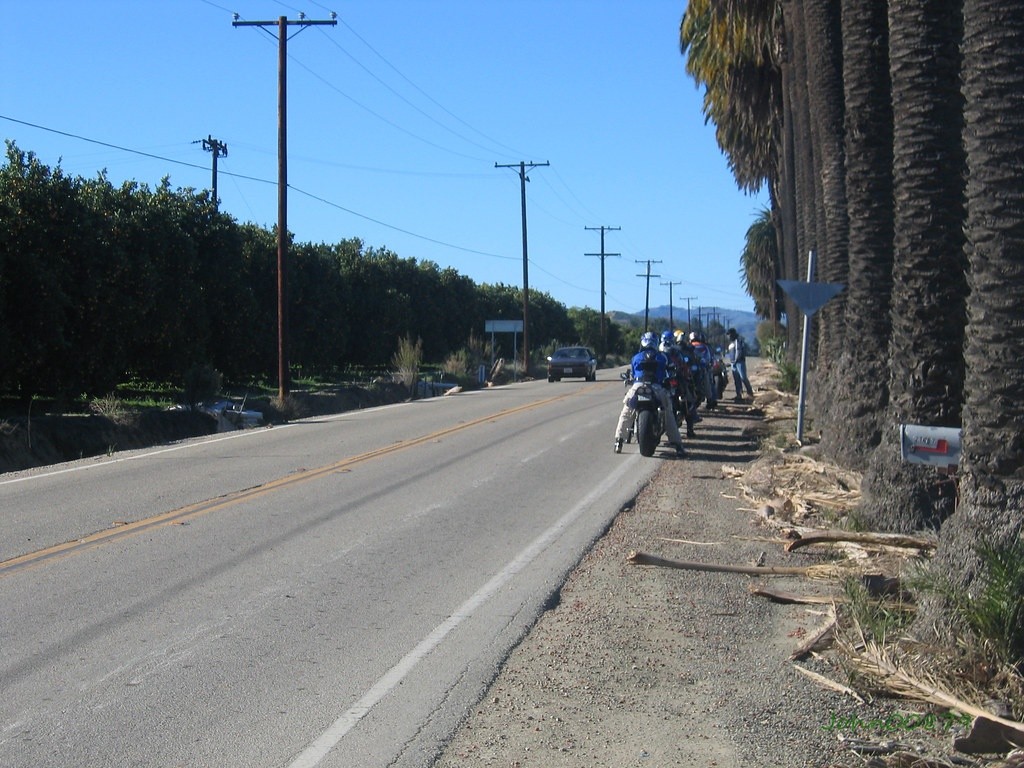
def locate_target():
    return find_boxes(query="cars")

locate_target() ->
[547,347,598,382]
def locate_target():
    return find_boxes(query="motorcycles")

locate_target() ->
[620,347,731,457]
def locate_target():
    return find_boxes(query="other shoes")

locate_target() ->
[614,441,622,453]
[685,431,697,439]
[692,416,701,423]
[745,395,752,399]
[707,402,717,409]
[675,450,692,460]
[732,395,742,399]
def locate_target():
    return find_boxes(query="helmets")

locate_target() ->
[640,332,660,349]
[672,330,685,341]
[688,332,699,342]
[660,330,673,346]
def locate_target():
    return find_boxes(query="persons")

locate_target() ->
[613,332,688,457]
[689,332,718,411]
[660,331,697,438]
[726,328,753,400]
[674,330,703,423]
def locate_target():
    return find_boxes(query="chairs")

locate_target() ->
[558,353,567,360]
